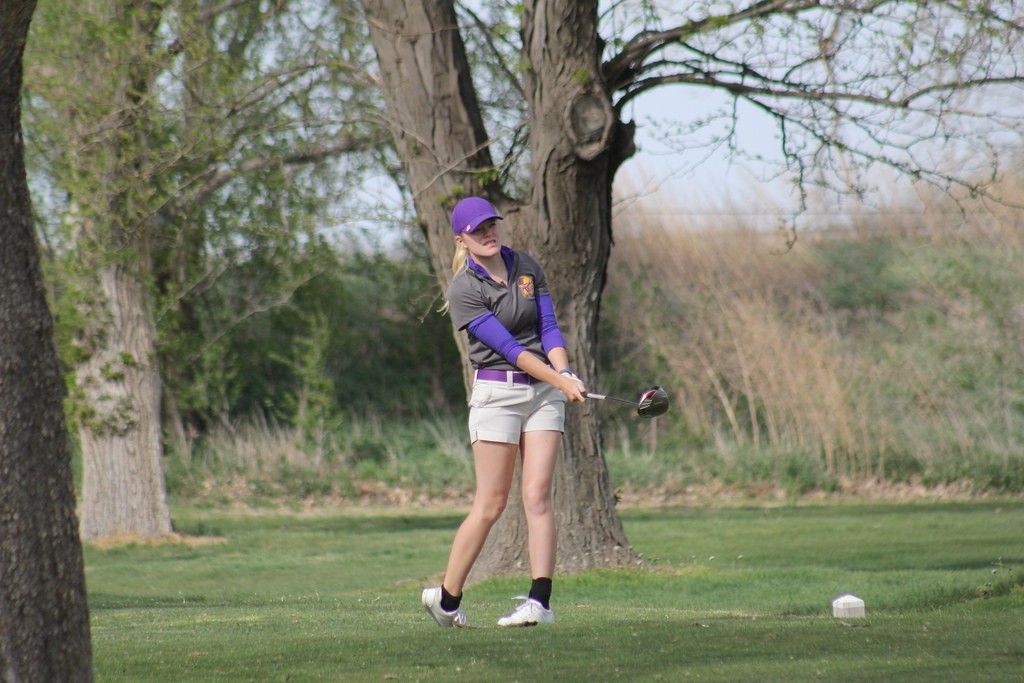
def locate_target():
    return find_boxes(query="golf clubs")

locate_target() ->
[581,387,670,419]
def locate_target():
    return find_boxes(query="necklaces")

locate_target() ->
[500,282,505,285]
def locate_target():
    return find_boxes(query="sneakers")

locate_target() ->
[423,588,466,630]
[497,595,554,626]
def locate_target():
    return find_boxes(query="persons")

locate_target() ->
[421,197,587,626]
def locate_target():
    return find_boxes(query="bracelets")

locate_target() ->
[559,368,571,375]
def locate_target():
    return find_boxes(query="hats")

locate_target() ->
[451,197,503,234]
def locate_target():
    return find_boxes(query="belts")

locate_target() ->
[476,365,556,385]
